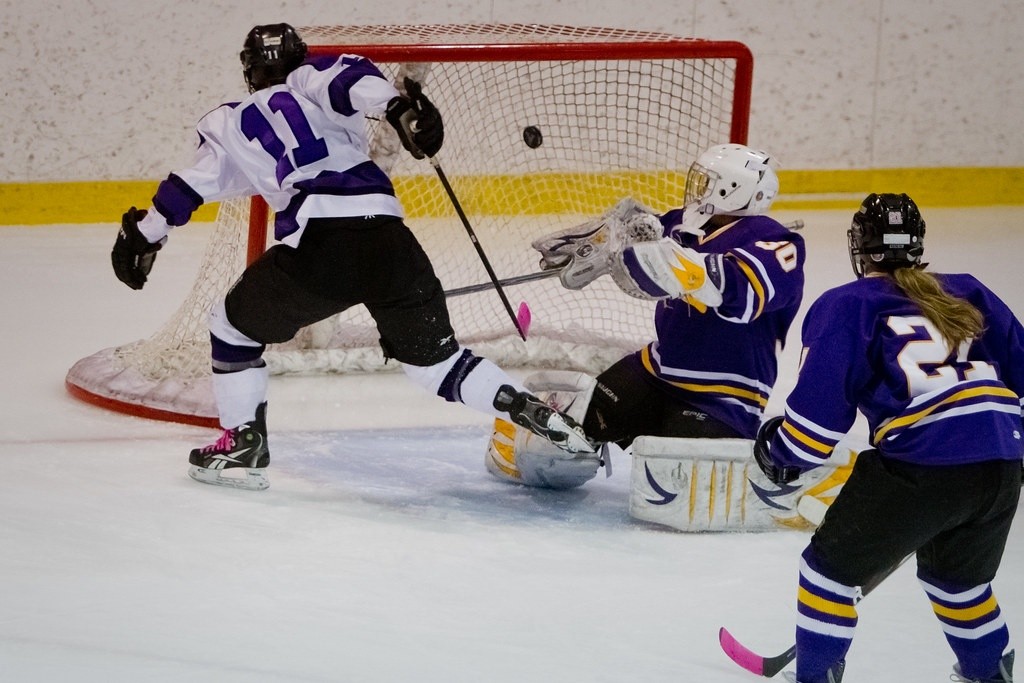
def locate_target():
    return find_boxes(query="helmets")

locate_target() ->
[851,192,927,273]
[682,144,781,233]
[240,23,309,88]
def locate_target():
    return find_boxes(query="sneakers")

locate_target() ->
[188,400,270,491]
[782,660,847,683]
[493,384,596,453]
[950,649,1015,683]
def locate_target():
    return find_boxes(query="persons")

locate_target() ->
[754,192,1024,683]
[112,22,596,493]
[531,144,806,492]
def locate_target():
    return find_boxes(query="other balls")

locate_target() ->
[523,126,545,149]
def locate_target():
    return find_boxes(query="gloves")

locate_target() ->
[385,92,443,160]
[111,207,168,290]
[747,416,850,511]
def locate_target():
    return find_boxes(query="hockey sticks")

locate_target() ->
[443,219,806,299]
[719,548,916,678]
[402,76,532,341]
